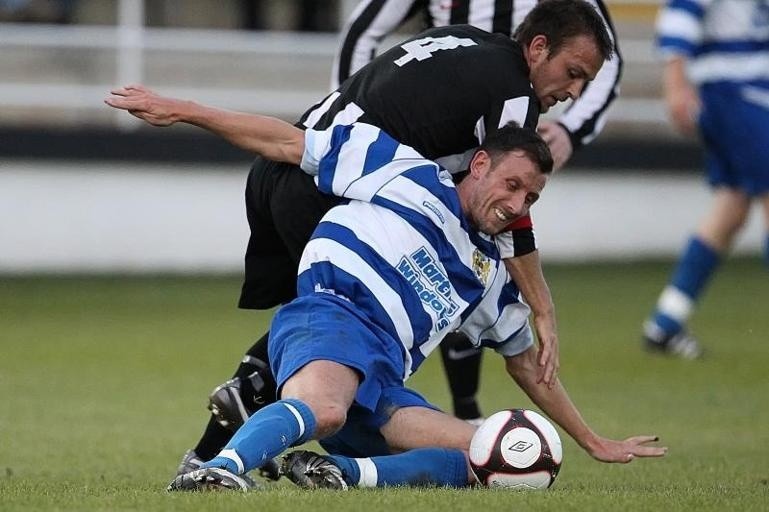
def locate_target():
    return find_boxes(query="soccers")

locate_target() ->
[469,409,563,490]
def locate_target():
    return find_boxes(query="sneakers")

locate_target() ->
[166,371,348,493]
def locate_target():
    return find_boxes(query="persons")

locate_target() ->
[175,0,612,480]
[640,0,769,360]
[329,0,624,426]
[104,83,670,491]
